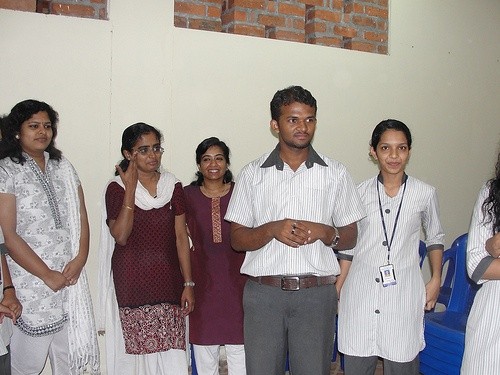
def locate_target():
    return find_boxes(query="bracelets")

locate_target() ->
[121,204,134,210]
[3,286,14,294]
[183,282,195,287]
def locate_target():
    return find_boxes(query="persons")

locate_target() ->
[110,84,500,375]
[0,99,101,375]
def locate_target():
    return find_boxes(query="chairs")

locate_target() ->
[418,233,483,375]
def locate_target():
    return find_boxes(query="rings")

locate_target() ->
[292,229,295,234]
[293,222,297,228]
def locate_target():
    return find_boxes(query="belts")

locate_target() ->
[247,275,337,292]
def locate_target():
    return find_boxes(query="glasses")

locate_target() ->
[131,146,165,155]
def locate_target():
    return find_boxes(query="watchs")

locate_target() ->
[323,225,340,248]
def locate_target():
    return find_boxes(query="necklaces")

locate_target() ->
[203,180,227,197]
